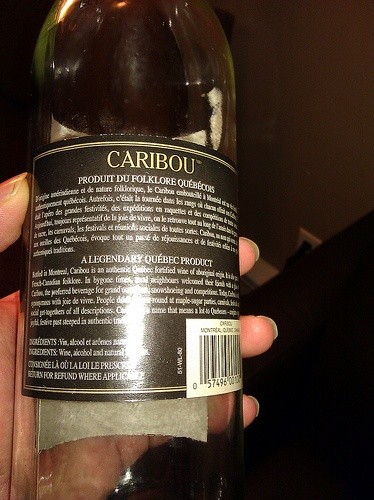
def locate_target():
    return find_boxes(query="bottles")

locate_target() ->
[19,2,253,500]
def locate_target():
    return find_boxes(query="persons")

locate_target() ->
[0,170,279,500]
